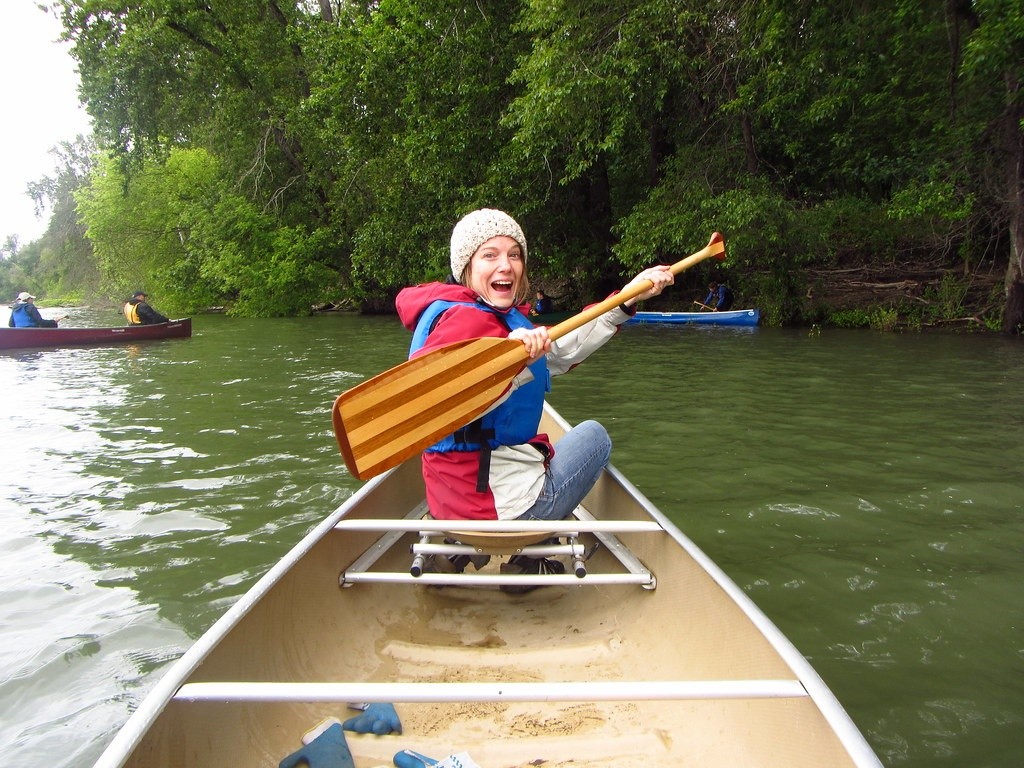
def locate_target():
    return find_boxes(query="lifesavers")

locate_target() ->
[747,312,755,316]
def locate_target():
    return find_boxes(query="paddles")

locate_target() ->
[333,228,726,483]
[55,315,70,326]
[692,298,719,311]
[167,320,181,322]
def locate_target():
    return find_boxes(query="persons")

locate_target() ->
[123,291,172,326]
[407,206,677,595]
[530,289,554,317]
[699,280,735,312]
[8,291,58,328]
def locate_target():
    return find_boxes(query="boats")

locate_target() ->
[625,307,760,326]
[0,316,193,350]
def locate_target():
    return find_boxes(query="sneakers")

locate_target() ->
[421,553,461,577]
[499,558,565,594]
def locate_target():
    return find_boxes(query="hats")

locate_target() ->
[133,291,149,298]
[15,292,37,301]
[449,208,528,283]
